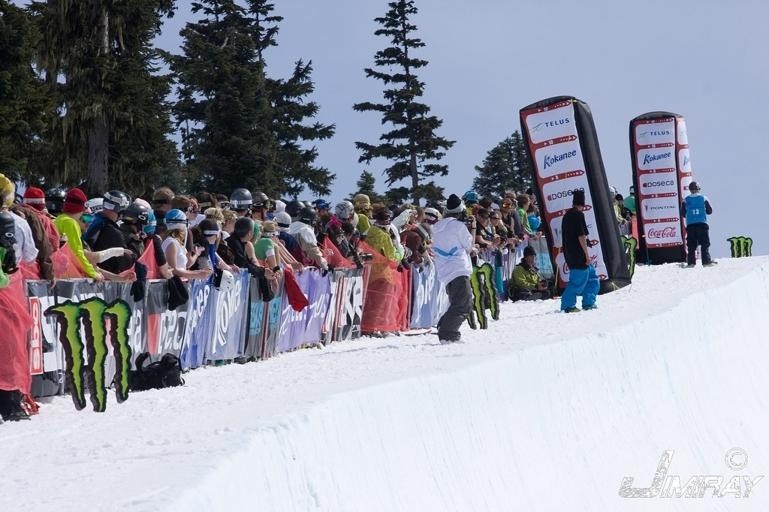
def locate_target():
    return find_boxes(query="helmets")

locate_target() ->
[165,209,188,231]
[463,191,478,203]
[336,201,359,227]
[106,192,157,233]
[286,200,316,223]
[230,188,270,212]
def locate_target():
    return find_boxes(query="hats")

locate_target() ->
[0,174,16,209]
[200,219,218,236]
[355,193,371,211]
[524,246,535,256]
[24,186,46,209]
[573,191,584,205]
[64,188,89,214]
[447,193,463,213]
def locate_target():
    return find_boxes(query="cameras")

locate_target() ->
[461,217,473,225]
[498,236,507,244]
[480,244,487,248]
[359,253,373,261]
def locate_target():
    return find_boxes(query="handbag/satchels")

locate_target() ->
[127,352,183,392]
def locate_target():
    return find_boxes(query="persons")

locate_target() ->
[680,181,717,268]
